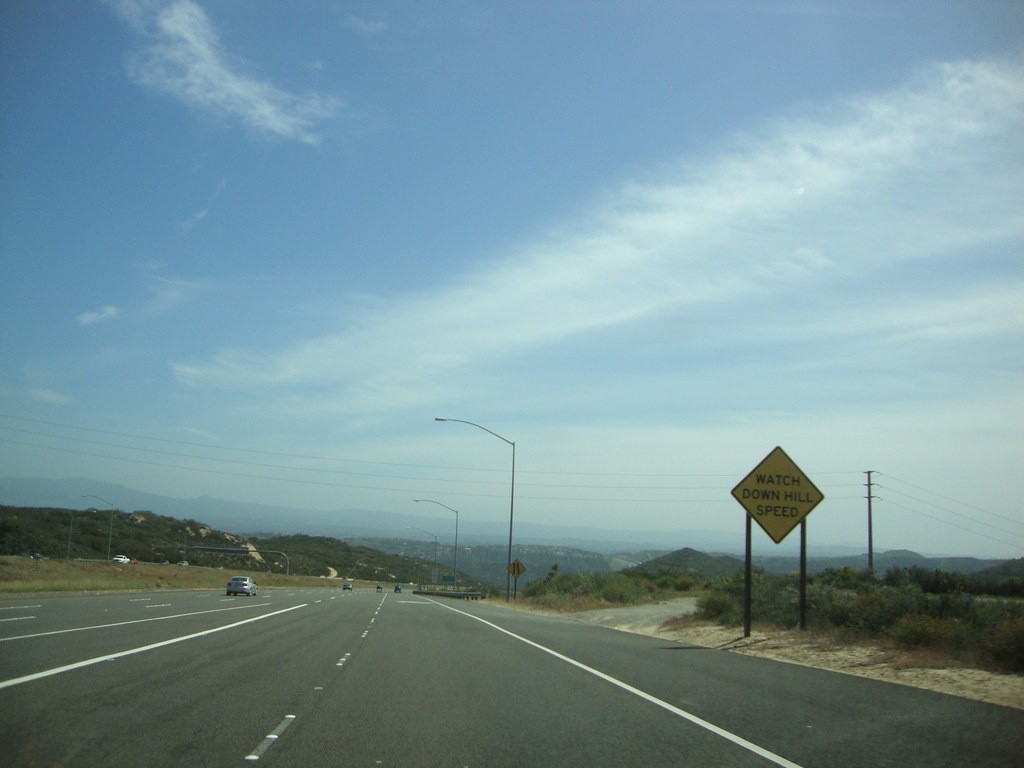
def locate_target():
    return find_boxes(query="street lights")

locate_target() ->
[412,498,458,592]
[406,526,438,590]
[82,494,114,562]
[434,417,516,602]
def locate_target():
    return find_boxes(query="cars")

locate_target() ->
[112,555,139,564]
[226,575,257,597]
[343,582,354,590]
[177,560,189,567]
